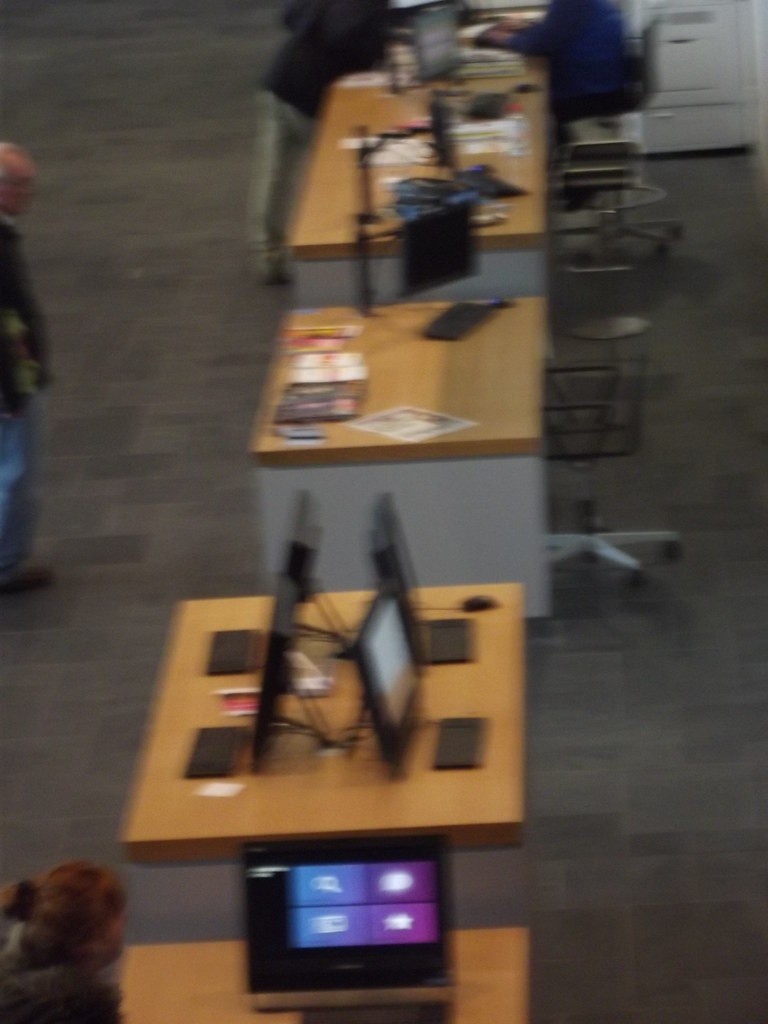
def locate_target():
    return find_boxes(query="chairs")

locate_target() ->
[556,52,679,214]
[556,136,695,337]
[546,350,684,600]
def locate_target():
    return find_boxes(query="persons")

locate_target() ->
[248,0,392,289]
[0,859,128,1024]
[486,0,632,213]
[0,141,56,593]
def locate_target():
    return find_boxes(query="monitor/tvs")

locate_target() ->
[243,488,424,768]
[350,5,482,319]
[239,836,455,994]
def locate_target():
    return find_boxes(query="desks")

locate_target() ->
[290,71,550,308]
[121,915,534,1021]
[115,584,531,864]
[246,294,555,622]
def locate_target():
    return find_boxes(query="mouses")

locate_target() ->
[463,594,497,612]
[485,301,506,310]
[519,87,539,95]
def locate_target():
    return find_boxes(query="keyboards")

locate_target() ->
[461,173,525,200]
[424,618,477,665]
[474,28,504,49]
[206,629,259,675]
[433,717,483,769]
[464,91,508,121]
[183,727,244,779]
[424,300,490,342]
